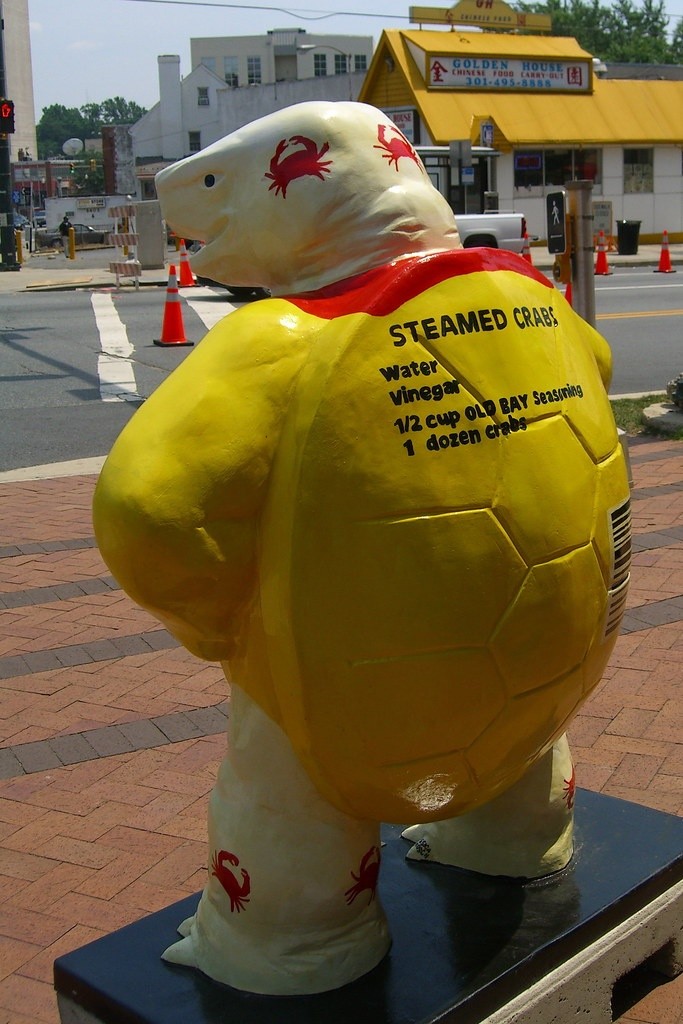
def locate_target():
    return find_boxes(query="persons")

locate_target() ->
[58,216,72,258]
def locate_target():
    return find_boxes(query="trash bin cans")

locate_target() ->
[615,219,641,256]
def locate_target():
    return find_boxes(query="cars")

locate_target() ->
[14,213,29,230]
[45,223,111,248]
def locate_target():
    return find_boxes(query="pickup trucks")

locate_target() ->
[200,213,527,298]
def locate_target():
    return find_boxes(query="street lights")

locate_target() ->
[57,176,62,196]
[296,43,352,102]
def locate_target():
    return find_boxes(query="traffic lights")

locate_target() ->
[70,163,74,174]
[0,99,16,135]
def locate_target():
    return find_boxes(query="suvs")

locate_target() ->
[33,210,47,228]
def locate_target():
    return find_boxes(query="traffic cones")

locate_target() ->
[153,263,194,348]
[178,239,200,287]
[593,231,611,275]
[653,230,677,274]
[566,282,573,309]
[522,233,532,265]
